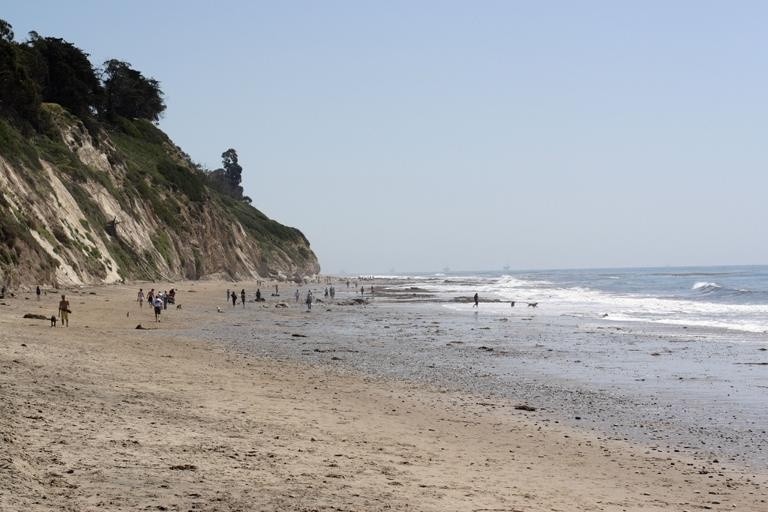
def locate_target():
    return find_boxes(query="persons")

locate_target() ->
[50,314,56,326]
[241,288,246,303]
[227,288,230,301]
[58,294,69,327]
[136,288,175,323]
[256,288,261,301]
[472,292,478,307]
[295,285,374,309]
[230,291,237,306]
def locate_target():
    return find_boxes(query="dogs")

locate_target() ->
[51,315,56,327]
[527,302,540,308]
